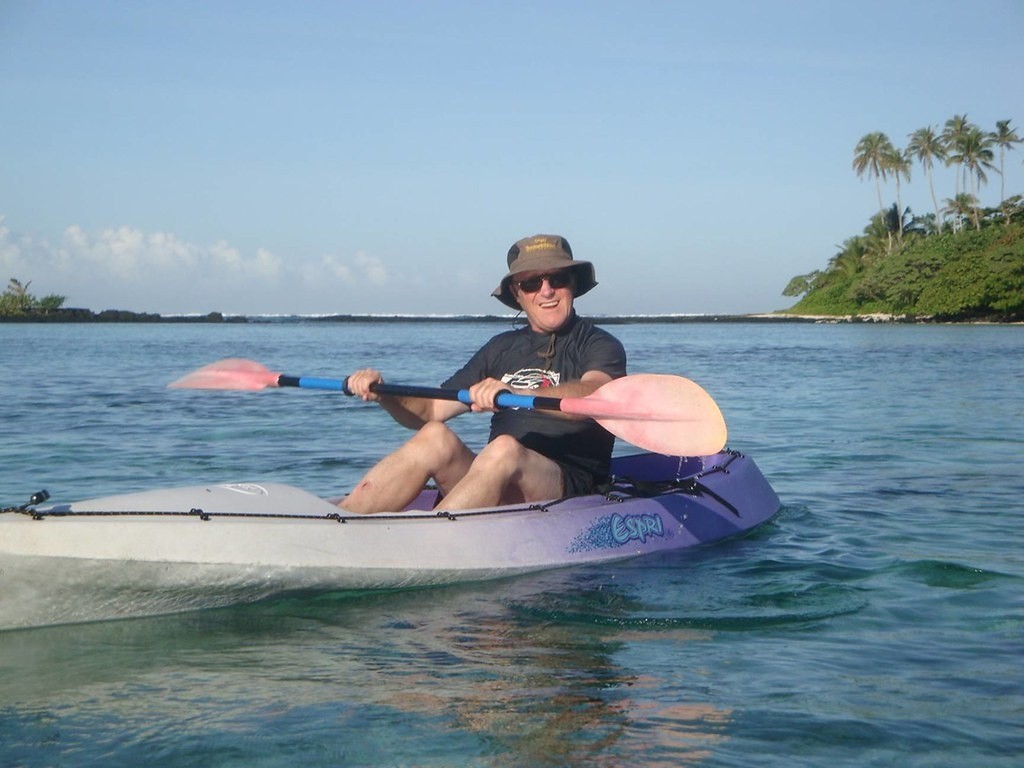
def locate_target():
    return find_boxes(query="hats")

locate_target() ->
[491,234,599,311]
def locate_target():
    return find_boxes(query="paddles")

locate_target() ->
[161,356,730,461]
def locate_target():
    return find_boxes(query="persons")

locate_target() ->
[335,235,626,514]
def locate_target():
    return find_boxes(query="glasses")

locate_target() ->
[513,270,572,293]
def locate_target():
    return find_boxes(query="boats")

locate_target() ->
[0,447,783,634]
